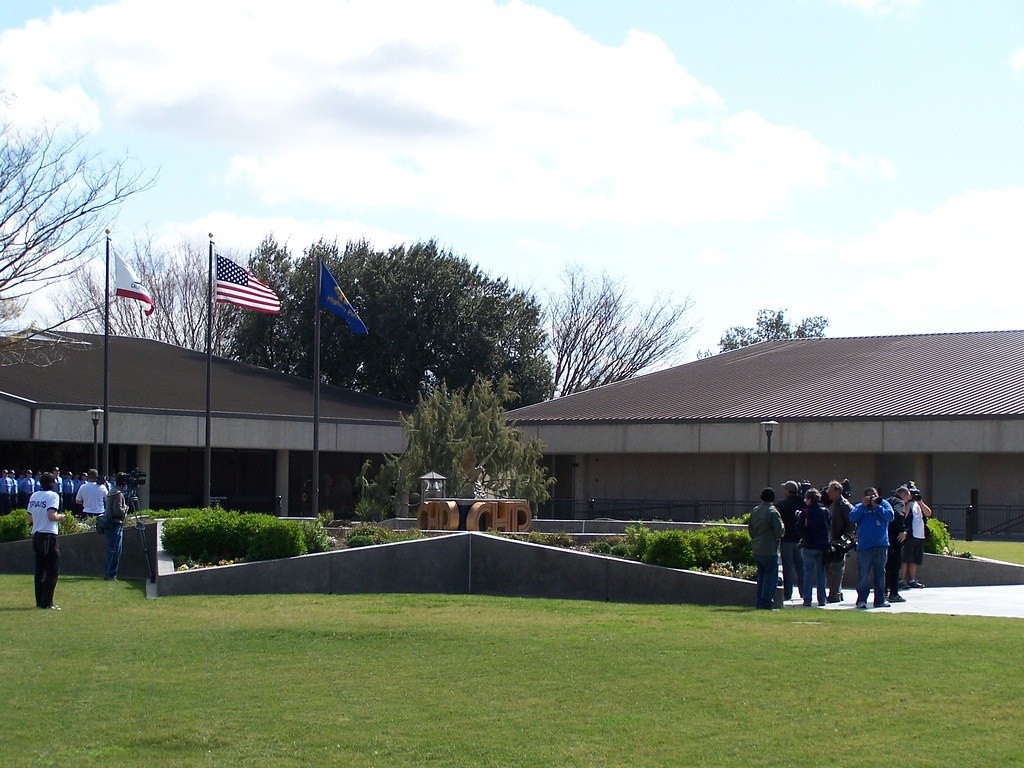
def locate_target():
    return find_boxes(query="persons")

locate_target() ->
[897,482,931,589]
[1,466,131,517]
[26,471,66,611]
[773,479,807,600]
[747,487,786,610]
[821,479,858,603]
[882,486,911,604]
[849,485,892,608]
[102,471,130,581]
[796,487,829,608]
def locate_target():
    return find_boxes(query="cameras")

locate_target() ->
[124,467,147,486]
[870,495,882,505]
[910,488,921,500]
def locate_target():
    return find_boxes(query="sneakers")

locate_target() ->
[908,578,926,588]
[876,602,891,607]
[897,579,910,590]
[889,593,906,603]
[855,600,868,608]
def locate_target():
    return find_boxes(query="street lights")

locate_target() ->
[760,416,778,488]
[87,405,103,469]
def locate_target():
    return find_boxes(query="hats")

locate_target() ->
[780,480,798,493]
[760,486,776,502]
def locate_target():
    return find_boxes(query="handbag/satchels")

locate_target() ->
[94,511,107,536]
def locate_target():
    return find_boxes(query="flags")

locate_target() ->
[318,258,370,338]
[112,247,155,316]
[213,252,282,315]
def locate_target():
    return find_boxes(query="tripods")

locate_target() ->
[104,490,156,584]
